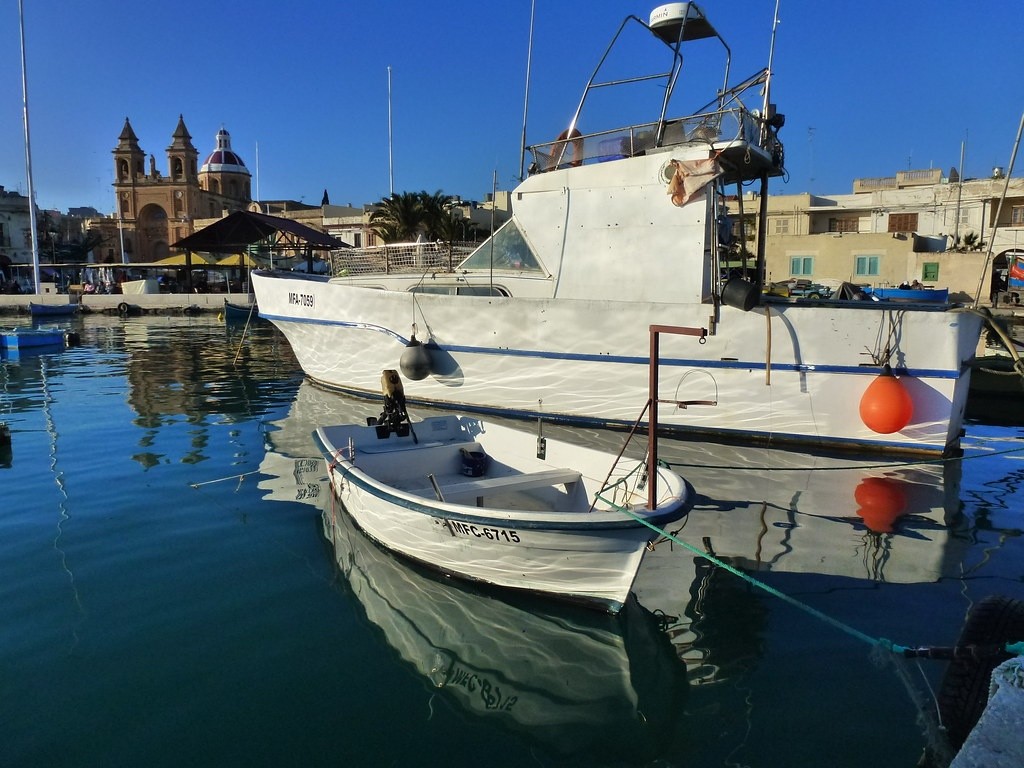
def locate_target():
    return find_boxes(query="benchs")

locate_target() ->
[406,466,582,503]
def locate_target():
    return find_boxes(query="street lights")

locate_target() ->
[386,65,393,197]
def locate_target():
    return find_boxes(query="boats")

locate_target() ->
[0,323,66,350]
[256,376,967,586]
[315,476,693,759]
[29,300,79,317]
[254,0,1023,459]
[309,365,697,616]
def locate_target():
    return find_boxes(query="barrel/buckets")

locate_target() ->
[720,274,760,312]
[461,451,486,477]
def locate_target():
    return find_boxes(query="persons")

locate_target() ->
[911,280,924,290]
[51,272,71,294]
[899,280,911,290]
[79,280,114,296]
[11,281,21,293]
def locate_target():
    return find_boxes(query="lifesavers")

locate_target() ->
[118,303,129,313]
[184,308,192,316]
